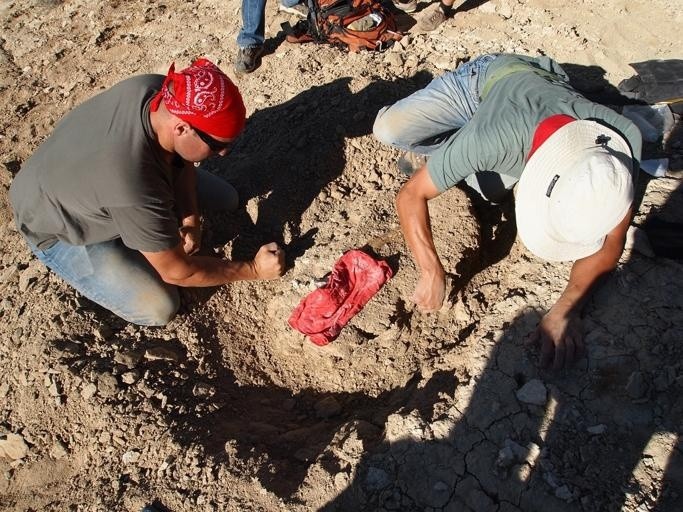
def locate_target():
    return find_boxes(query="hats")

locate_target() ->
[515,119,635,262]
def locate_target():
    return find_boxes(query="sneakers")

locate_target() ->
[236,46,265,73]
[393,0,417,13]
[419,6,451,31]
[280,4,309,17]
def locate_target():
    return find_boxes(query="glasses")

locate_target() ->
[192,126,231,151]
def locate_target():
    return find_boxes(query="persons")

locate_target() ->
[8,58,285,327]
[372,52,644,367]
[234,0,307,73]
[393,0,457,32]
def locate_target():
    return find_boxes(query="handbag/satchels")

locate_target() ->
[315,0,396,48]
